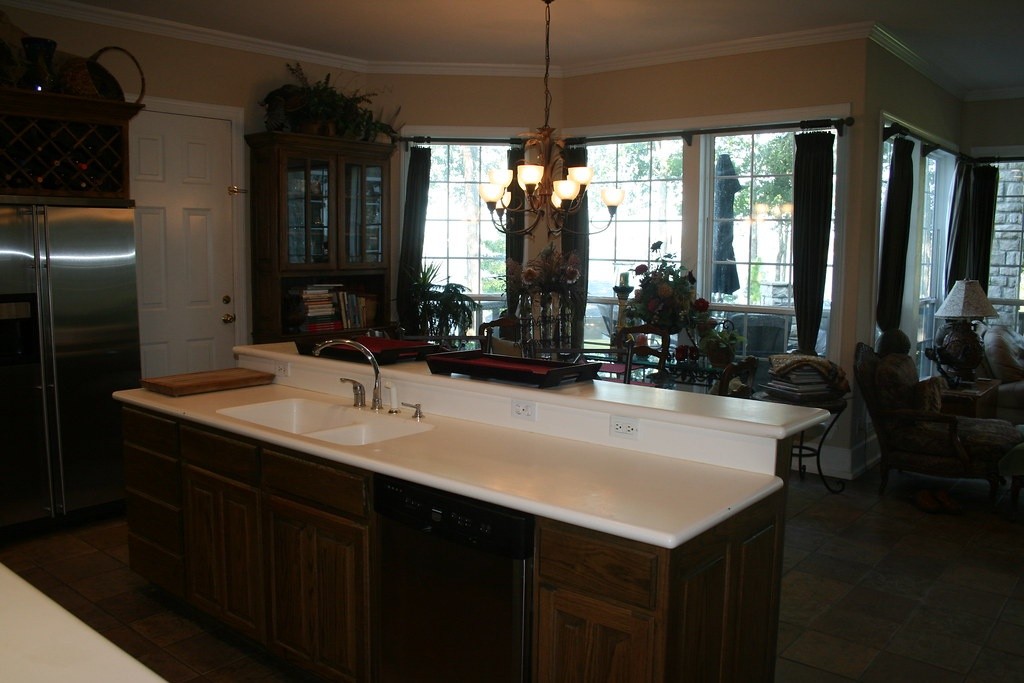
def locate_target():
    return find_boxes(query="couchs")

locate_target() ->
[969,319,1023,422]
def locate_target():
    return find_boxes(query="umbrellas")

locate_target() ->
[710,154,744,303]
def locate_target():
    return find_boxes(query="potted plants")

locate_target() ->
[259,59,407,145]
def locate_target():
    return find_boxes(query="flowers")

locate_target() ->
[504,241,749,370]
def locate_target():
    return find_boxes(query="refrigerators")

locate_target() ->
[0,194,141,528]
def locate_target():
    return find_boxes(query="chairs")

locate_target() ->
[856,342,1024,520]
[725,314,792,357]
[709,356,757,398]
[479,318,526,357]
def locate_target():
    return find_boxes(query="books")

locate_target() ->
[758,364,830,396]
[287,283,367,333]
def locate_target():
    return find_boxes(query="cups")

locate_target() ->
[675,344,699,364]
[287,175,381,263]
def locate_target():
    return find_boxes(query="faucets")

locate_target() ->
[384,382,401,414]
[312,338,383,410]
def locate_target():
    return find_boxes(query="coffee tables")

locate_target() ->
[750,390,845,494]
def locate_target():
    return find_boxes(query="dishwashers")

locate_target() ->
[373,473,534,683]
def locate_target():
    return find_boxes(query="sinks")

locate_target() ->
[216,399,360,434]
[301,423,435,446]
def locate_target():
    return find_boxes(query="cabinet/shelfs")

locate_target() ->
[179,423,260,648]
[121,405,185,601]
[259,450,372,683]
[534,527,653,682]
[244,130,395,347]
[938,374,1000,418]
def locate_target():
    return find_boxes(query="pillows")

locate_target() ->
[916,375,943,426]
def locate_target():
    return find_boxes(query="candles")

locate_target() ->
[619,272,629,287]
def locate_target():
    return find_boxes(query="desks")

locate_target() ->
[535,354,720,395]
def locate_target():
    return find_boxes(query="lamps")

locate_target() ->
[478,0,625,236]
[933,279,999,328]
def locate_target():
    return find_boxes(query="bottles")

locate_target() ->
[3,142,97,190]
